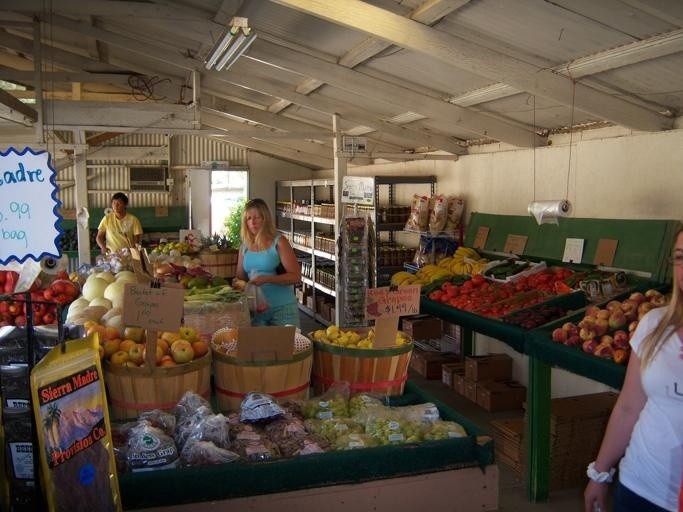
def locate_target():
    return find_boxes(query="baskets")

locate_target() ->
[179,293,253,349]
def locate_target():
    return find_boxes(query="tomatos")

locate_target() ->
[429,268,574,322]
[249,303,267,312]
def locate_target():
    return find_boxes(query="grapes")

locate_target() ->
[302,392,467,451]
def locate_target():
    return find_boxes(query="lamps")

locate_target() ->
[203,16,257,71]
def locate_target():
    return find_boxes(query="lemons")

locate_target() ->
[315,324,411,352]
[153,242,188,255]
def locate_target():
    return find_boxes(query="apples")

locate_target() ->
[84,321,207,367]
[235,279,247,292]
[0,270,86,328]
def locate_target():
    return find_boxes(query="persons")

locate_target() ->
[231,198,300,334]
[582,226,683,511]
[95,192,143,257]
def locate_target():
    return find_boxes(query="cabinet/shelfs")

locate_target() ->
[530,219,683,504]
[273,175,436,329]
[407,211,668,504]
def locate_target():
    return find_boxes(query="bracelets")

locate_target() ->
[586,460,616,483]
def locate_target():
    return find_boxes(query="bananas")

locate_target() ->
[389,245,491,288]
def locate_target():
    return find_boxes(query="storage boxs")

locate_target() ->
[401,317,621,490]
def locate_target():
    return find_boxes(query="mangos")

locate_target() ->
[214,326,312,359]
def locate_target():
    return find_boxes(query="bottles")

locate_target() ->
[276,199,419,292]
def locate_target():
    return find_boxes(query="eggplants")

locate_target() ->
[163,262,229,290]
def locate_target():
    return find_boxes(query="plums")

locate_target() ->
[504,306,568,330]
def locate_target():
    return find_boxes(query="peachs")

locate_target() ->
[552,288,672,364]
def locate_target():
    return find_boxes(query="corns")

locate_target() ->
[183,285,233,304]
